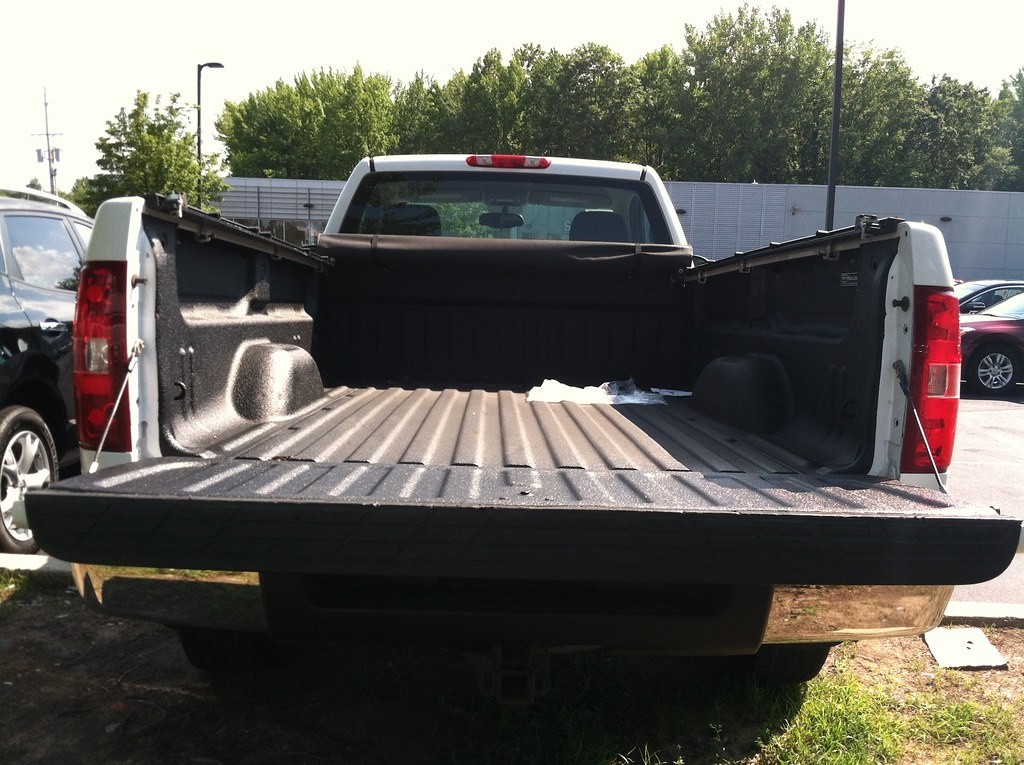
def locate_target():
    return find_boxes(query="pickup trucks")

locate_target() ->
[18,150,1024,679]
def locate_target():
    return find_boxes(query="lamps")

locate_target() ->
[940,217,952,222]
[303,204,314,208]
[676,209,686,213]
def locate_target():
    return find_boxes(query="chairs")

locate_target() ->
[568,211,627,242]
[382,204,440,236]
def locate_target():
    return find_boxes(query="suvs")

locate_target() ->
[0,184,97,554]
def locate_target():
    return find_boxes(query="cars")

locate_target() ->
[959,292,1024,393]
[954,277,1024,314]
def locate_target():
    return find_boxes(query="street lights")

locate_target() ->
[197,60,223,208]
